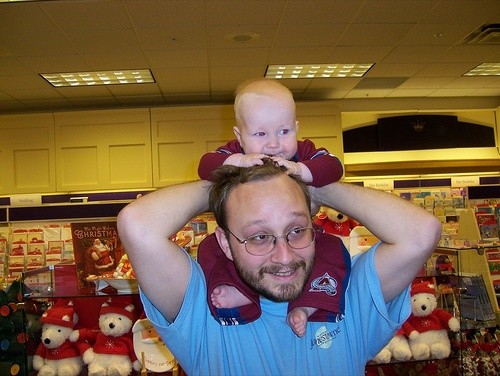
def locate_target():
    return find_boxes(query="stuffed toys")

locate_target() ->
[403,281,460,363]
[312,205,363,257]
[32,302,96,376]
[69,296,143,376]
[371,323,412,363]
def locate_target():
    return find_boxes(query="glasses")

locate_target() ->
[225,226,316,256]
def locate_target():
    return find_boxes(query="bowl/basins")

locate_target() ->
[104,280,139,292]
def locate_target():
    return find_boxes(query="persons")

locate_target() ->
[194,78,352,339]
[90,238,114,278]
[117,156,443,376]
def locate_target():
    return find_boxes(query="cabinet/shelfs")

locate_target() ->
[459,208,500,312]
